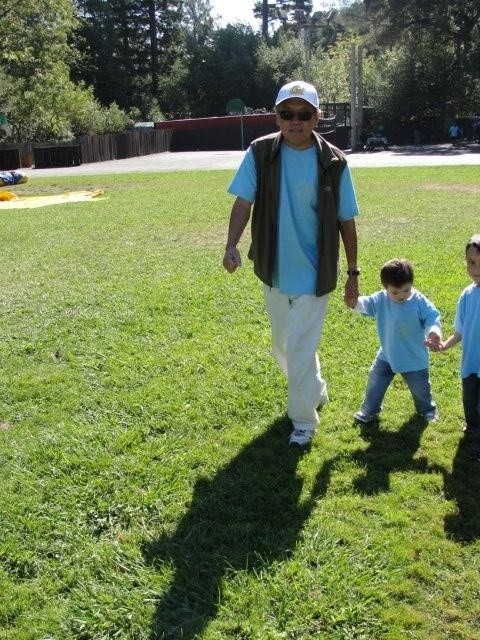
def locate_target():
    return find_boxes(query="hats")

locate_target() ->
[276,81,319,112]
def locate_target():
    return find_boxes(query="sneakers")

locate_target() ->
[288,428,316,447]
[353,410,378,422]
[424,408,438,424]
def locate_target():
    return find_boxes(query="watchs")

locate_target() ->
[346,268,362,276]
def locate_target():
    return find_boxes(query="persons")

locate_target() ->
[423,232,479,434]
[342,258,444,430]
[448,122,462,146]
[222,81,361,449]
[471,115,479,143]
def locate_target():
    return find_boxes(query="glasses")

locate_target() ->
[277,108,315,121]
